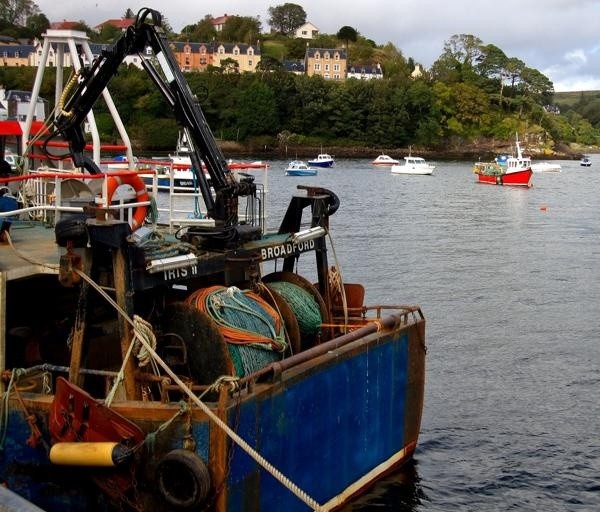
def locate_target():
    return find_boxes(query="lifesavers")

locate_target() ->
[96,170,148,231]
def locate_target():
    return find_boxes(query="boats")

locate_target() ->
[580,159,592,167]
[391,154,435,175]
[474,131,561,187]
